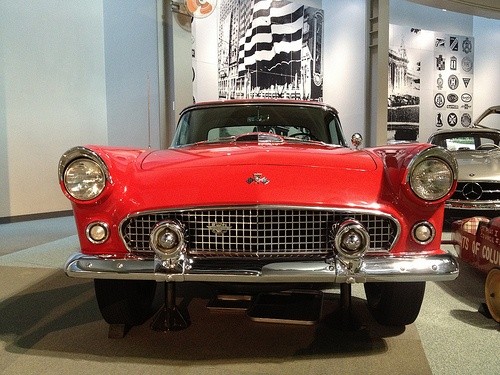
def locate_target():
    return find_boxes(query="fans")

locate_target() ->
[183,0,218,19]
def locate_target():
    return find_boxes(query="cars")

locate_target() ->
[56,98,455,330]
[426,128,500,213]
